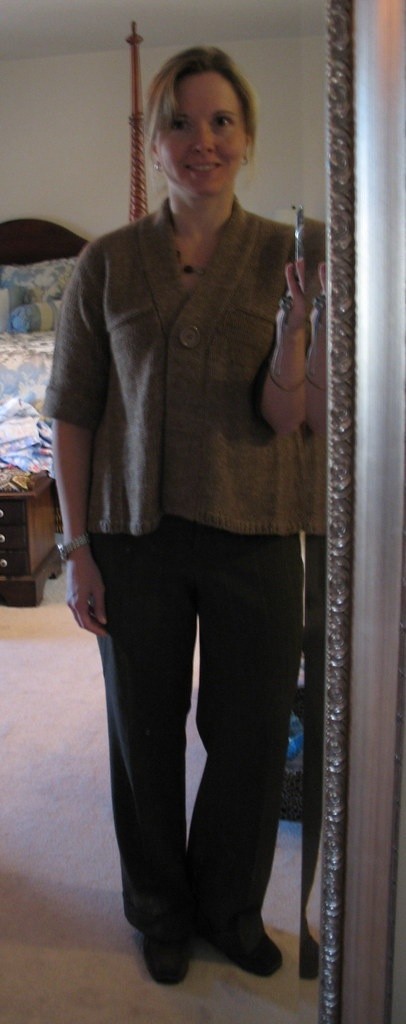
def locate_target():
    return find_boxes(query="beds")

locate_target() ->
[0,218,92,480]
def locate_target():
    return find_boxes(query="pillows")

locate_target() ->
[11,299,60,331]
[0,256,78,305]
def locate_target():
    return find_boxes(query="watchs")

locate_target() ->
[57,533,90,561]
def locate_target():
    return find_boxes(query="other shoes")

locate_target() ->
[145,930,189,984]
[195,917,282,977]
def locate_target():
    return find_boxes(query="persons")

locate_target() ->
[40,47,328,986]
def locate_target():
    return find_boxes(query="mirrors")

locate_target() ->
[0,0,353,1024]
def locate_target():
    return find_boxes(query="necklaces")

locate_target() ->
[176,245,206,276]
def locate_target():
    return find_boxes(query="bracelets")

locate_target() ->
[267,366,305,393]
[306,375,328,392]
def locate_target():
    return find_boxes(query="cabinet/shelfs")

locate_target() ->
[0,477,62,607]
